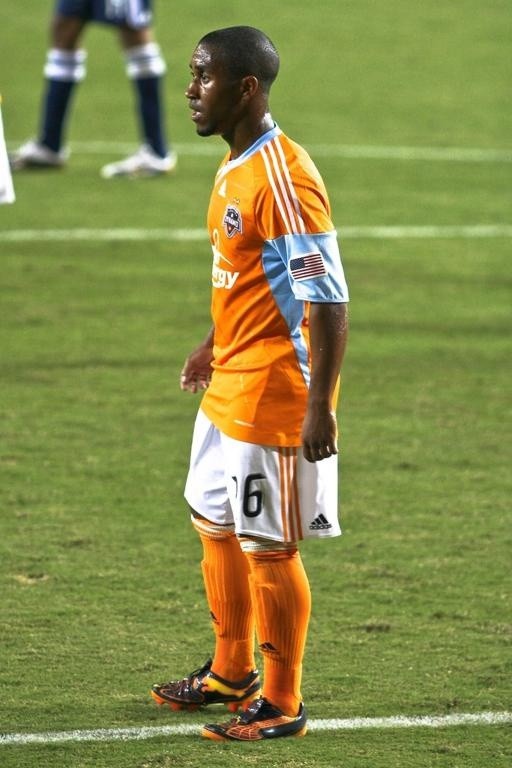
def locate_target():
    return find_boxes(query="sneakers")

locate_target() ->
[8,140,70,170]
[152,658,261,713]
[101,144,176,178]
[202,697,306,742]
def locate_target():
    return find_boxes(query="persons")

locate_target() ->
[148,23,351,745]
[8,0,179,180]
